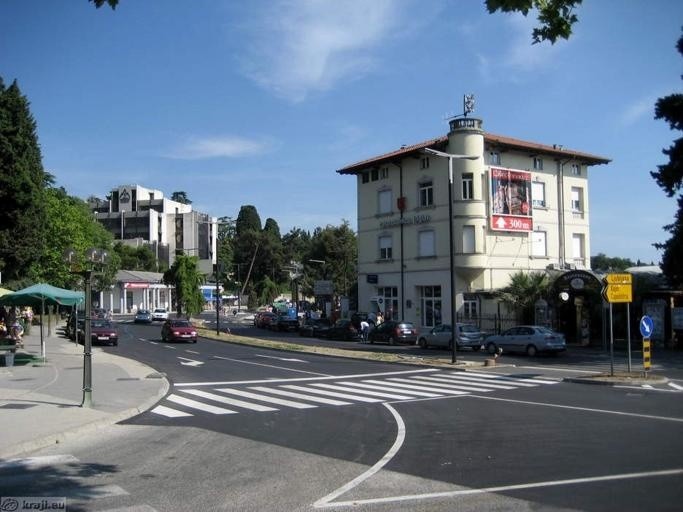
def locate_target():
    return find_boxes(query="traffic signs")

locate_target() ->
[602,286,633,304]
[602,271,632,285]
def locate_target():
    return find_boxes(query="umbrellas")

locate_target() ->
[1,281,86,363]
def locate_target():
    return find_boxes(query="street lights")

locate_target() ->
[420,146,481,366]
[198,218,233,336]
[59,246,110,407]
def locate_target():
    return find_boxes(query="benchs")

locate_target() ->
[0,338,16,366]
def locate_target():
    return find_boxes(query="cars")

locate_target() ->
[151,308,167,321]
[134,309,153,323]
[64,308,117,347]
[324,318,370,342]
[368,320,417,347]
[161,318,198,343]
[415,322,483,352]
[479,325,567,356]
[252,305,298,333]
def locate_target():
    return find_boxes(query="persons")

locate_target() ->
[360,310,384,344]
[0,304,35,341]
